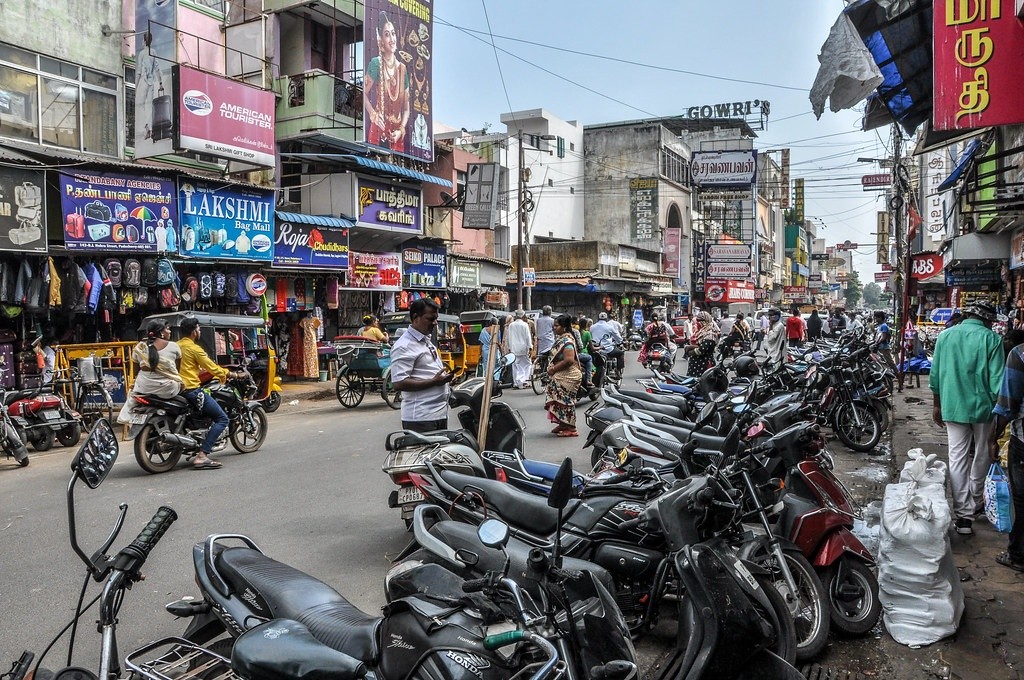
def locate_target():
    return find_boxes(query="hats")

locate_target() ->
[963,299,998,320]
[599,312,608,318]
[516,309,525,317]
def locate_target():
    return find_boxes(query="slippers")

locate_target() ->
[194,459,223,467]
[551,425,579,437]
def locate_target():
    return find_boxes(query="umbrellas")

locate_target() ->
[130,207,157,239]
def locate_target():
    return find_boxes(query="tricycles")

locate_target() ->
[333,336,452,410]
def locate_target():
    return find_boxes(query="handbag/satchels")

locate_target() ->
[211,230,218,243]
[85,200,112,222]
[184,227,195,251]
[905,321,916,340]
[117,397,138,423]
[35,344,46,369]
[982,463,1015,533]
[873,340,878,353]
[9,181,43,245]
[183,224,187,238]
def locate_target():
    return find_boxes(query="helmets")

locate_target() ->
[700,366,729,394]
[736,356,759,377]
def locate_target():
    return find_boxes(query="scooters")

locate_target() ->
[0,326,896,680]
[576,321,677,401]
[0,362,83,466]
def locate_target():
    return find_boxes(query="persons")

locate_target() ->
[360,314,389,342]
[116,318,249,469]
[391,297,455,435]
[478,305,555,386]
[544,311,625,436]
[299,312,322,377]
[849,307,1024,571]
[639,306,848,377]
[364,9,410,154]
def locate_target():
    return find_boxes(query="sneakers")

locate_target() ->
[974,507,989,525]
[953,518,972,533]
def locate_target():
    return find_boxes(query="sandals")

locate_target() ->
[995,552,1024,570]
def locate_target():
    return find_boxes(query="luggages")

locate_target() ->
[218,224,228,243]
[194,217,201,242]
[199,220,210,243]
[65,204,86,238]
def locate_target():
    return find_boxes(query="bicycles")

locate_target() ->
[46,367,113,434]
[526,349,549,395]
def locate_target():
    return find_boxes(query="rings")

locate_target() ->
[399,23,429,69]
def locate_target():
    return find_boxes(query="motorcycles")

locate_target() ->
[124,358,268,474]
[137,311,282,413]
[382,310,515,385]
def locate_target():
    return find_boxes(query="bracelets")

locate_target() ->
[370,110,376,122]
[398,125,406,140]
[234,373,237,379]
[835,325,837,328]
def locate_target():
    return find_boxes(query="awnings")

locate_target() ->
[847,0,933,138]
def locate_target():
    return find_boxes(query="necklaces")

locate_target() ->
[397,7,409,46]
[413,69,426,91]
[381,56,400,101]
[416,121,427,142]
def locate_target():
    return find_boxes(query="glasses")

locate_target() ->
[430,347,438,359]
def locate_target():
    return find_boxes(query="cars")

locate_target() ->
[670,317,689,348]
[755,309,769,333]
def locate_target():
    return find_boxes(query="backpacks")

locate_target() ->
[102,256,263,314]
[113,225,125,241]
[115,204,128,221]
